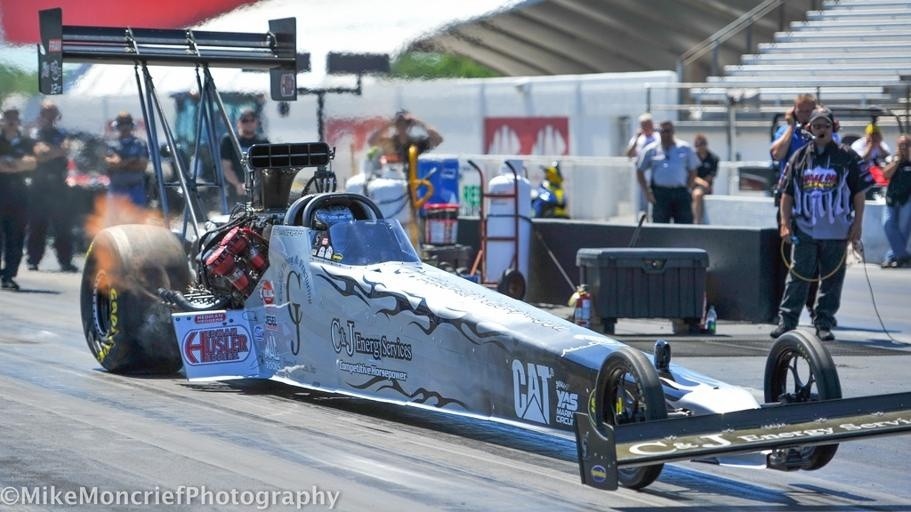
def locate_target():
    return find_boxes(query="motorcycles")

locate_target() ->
[842,135,897,201]
[531,160,571,219]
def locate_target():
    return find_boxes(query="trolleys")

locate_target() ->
[466,159,528,301]
[361,145,435,262]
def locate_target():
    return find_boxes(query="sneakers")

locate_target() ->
[27,263,38,271]
[0,278,20,292]
[816,325,835,342]
[61,264,79,273]
[770,320,796,339]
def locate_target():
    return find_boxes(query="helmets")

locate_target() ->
[310,205,356,261]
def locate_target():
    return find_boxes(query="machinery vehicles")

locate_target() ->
[147,88,301,223]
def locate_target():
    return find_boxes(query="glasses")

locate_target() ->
[241,118,256,123]
[813,123,832,129]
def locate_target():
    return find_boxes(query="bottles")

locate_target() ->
[706,305,717,334]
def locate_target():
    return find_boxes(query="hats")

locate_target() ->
[238,107,258,120]
[809,106,834,125]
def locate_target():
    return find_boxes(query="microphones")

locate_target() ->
[796,116,801,123]
[802,130,825,140]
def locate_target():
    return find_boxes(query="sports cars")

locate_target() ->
[38,8,911,491]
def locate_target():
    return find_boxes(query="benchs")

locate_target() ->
[689,1,911,124]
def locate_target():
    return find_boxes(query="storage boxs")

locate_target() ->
[574,246,711,320]
[422,242,478,277]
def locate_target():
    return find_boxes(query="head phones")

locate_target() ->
[792,93,816,123]
[110,112,137,130]
[804,108,840,133]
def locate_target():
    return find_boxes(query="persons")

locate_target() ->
[219,104,271,213]
[688,135,720,224]
[0,103,150,292]
[767,93,911,344]
[636,121,702,224]
[625,113,661,221]
[367,110,443,164]
